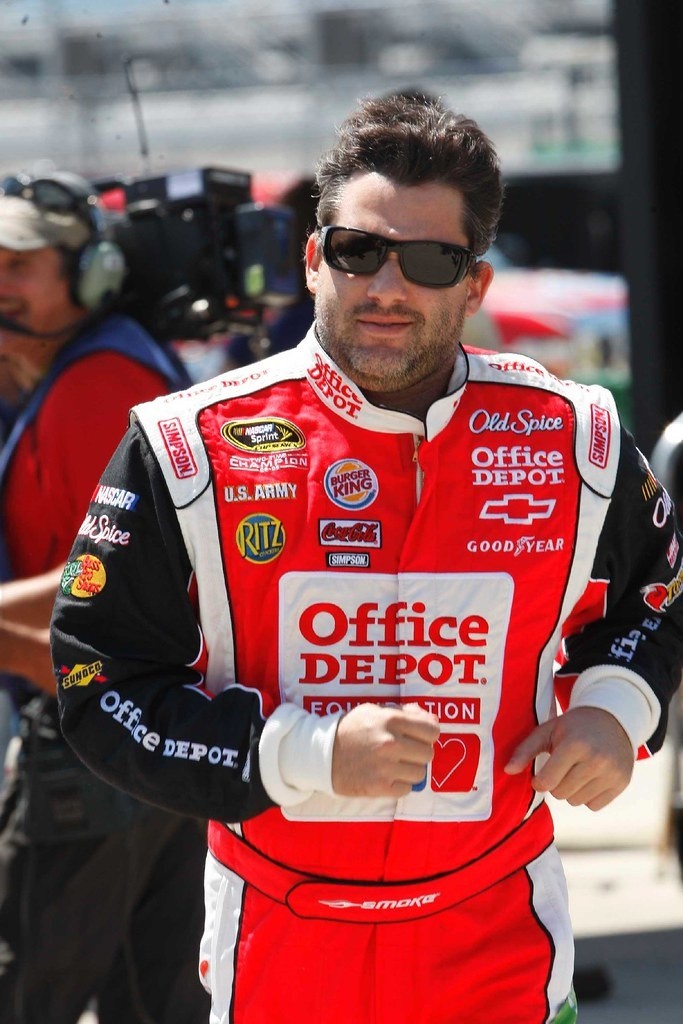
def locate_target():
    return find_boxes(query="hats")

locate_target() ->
[0,167,109,251]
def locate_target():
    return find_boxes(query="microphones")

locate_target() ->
[0,317,85,337]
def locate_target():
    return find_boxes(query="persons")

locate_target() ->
[0,86,683,1024]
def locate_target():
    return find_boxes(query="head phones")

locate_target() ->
[23,171,125,315]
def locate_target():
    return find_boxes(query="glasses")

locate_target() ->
[1,176,98,242]
[320,226,476,289]
